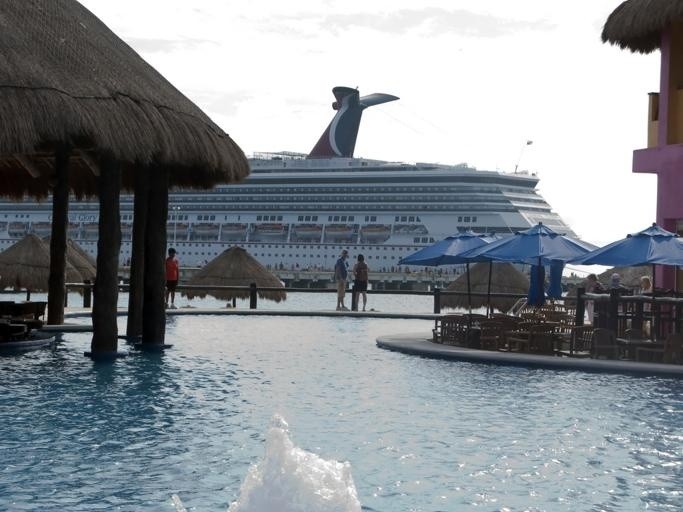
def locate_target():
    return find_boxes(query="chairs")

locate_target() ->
[553,325,594,357]
[577,288,683,338]
[636,334,683,363]
[433,304,576,355]
[589,328,618,360]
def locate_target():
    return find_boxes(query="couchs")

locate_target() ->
[0,302,48,320]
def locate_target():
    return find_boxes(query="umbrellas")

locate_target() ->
[456,222,596,306]
[396,231,494,315]
[566,222,683,298]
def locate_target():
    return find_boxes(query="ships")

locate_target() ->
[0,85,580,295]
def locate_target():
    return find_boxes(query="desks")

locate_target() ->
[11,320,45,337]
[616,338,667,359]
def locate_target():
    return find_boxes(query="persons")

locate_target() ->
[165,248,179,308]
[586,273,607,323]
[352,254,368,311]
[609,273,626,295]
[334,249,350,311]
[639,276,653,336]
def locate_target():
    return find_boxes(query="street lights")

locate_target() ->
[171,205,180,244]
